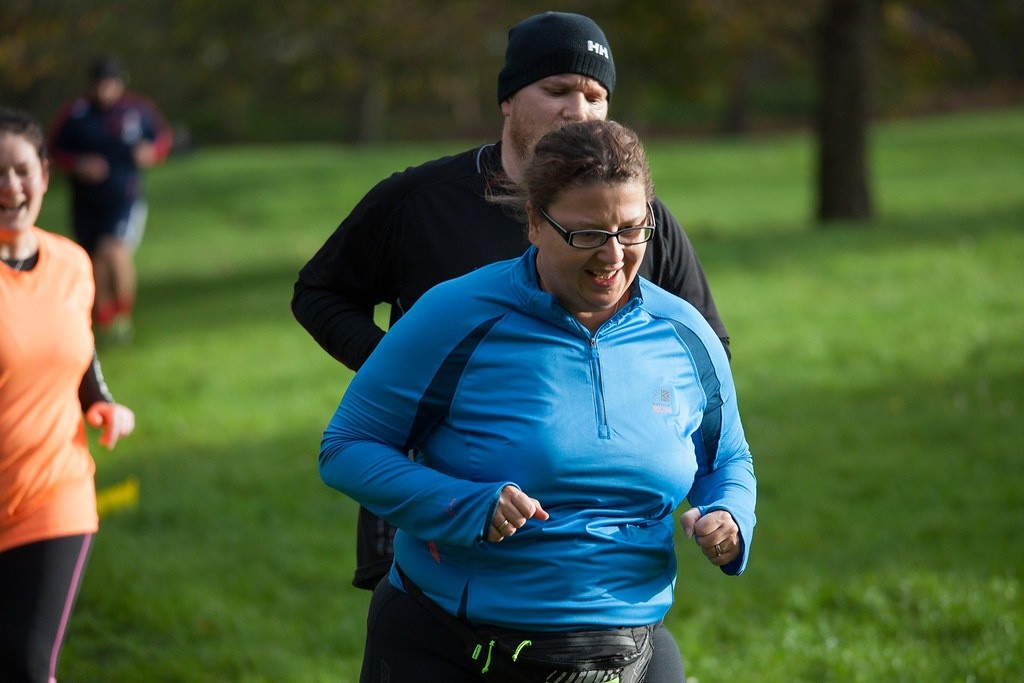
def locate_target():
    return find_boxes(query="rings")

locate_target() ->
[498,520,509,530]
[715,545,722,556]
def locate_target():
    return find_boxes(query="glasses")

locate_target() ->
[534,201,657,249]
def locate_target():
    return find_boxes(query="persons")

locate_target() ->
[0,111,134,683]
[317,119,757,683]
[47,58,171,334]
[291,12,731,593]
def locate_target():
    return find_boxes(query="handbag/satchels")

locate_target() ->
[458,619,655,683]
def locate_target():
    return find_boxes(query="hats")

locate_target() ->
[88,56,121,81]
[497,10,617,102]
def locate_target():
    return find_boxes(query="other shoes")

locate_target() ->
[96,313,132,338]
